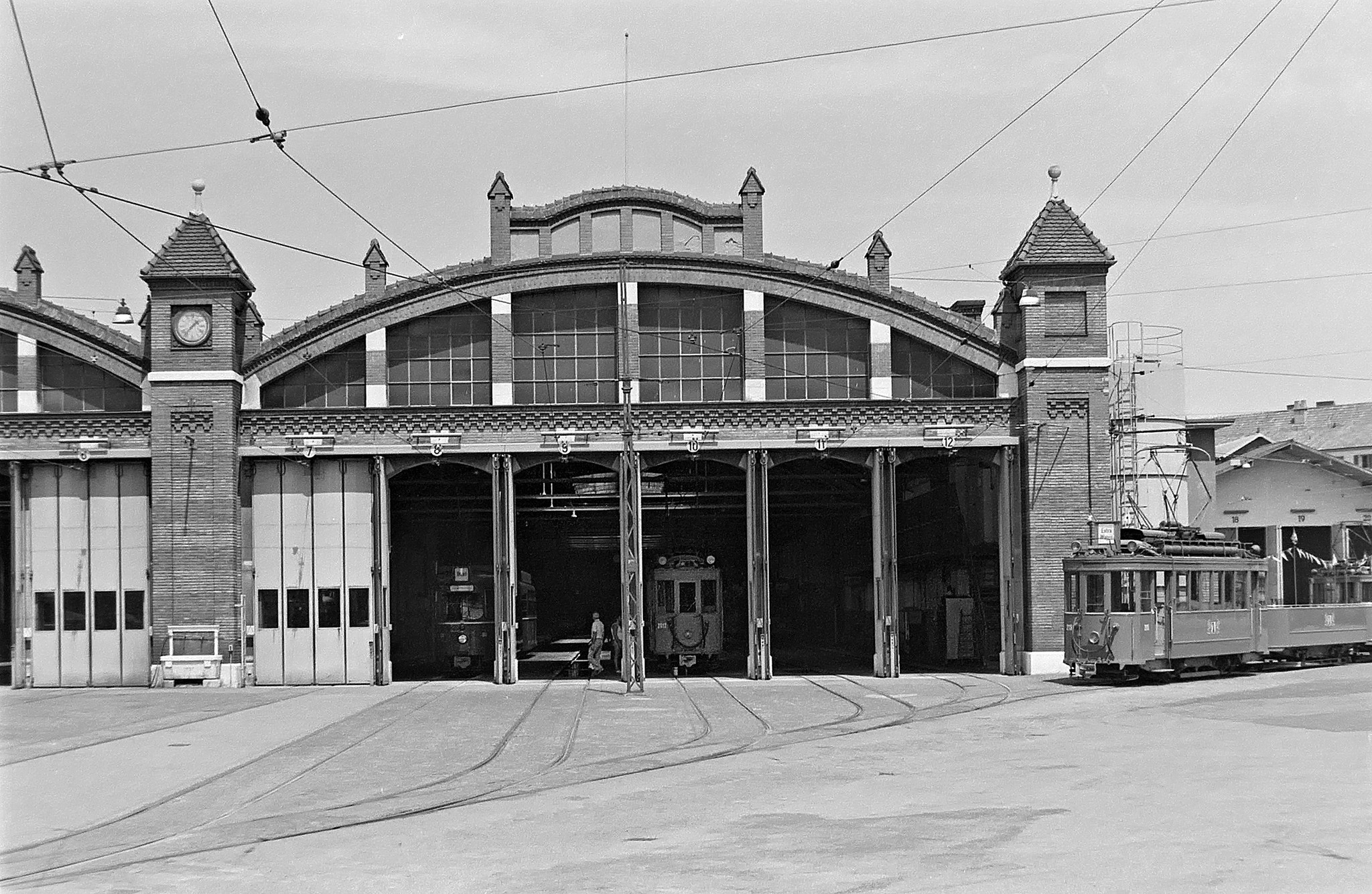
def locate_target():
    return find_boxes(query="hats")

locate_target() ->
[593,613,599,618]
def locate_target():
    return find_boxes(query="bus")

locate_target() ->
[643,549,725,675]
[1062,443,1372,687]
[428,559,539,677]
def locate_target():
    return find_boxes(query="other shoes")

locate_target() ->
[592,668,603,676]
[616,670,621,673]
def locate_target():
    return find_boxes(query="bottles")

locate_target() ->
[672,667,678,676]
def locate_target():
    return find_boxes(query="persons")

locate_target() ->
[588,612,623,677]
